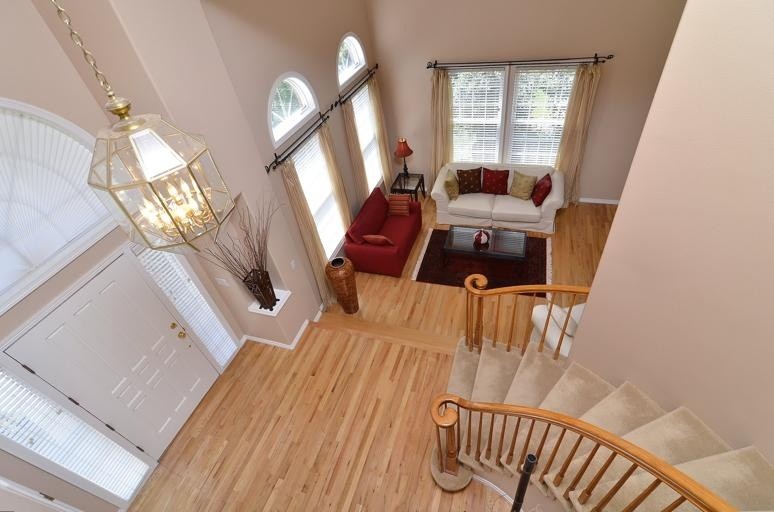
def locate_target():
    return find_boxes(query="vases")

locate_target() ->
[325,257,360,314]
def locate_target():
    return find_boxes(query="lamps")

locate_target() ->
[394,138,413,176]
[49,1,237,257]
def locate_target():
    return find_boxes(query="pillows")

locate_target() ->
[547,303,577,337]
[444,167,552,207]
[387,194,410,217]
[362,234,394,245]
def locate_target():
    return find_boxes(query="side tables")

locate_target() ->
[390,173,425,201]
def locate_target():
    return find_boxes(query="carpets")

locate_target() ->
[411,227,552,300]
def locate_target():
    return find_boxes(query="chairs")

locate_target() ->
[531,302,586,358]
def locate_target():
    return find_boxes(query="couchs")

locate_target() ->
[342,187,422,278]
[429,162,565,230]
